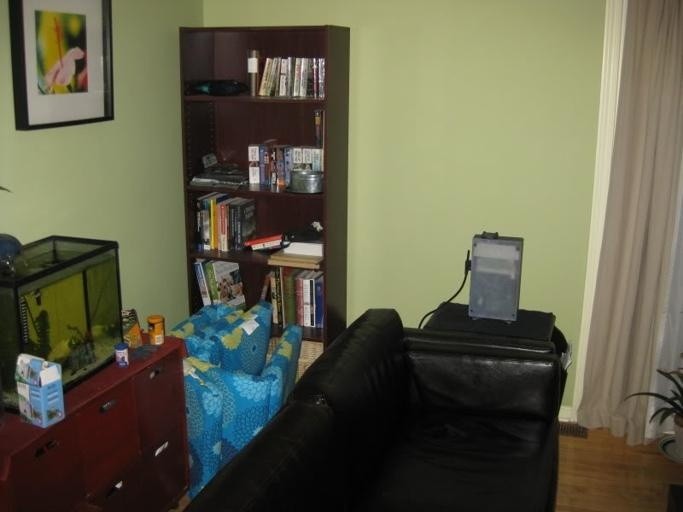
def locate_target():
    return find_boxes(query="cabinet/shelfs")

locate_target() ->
[0,334,189,509]
[178,25,351,347]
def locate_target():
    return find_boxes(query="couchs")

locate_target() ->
[184,307,568,511]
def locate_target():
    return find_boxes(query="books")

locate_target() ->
[194,192,323,328]
[247,50,325,100]
[248,139,323,186]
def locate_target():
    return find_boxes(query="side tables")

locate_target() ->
[426,302,570,415]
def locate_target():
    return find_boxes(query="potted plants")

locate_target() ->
[619,363,683,462]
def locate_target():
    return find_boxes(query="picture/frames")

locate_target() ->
[7,1,113,133]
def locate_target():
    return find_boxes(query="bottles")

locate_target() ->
[114,342,129,366]
